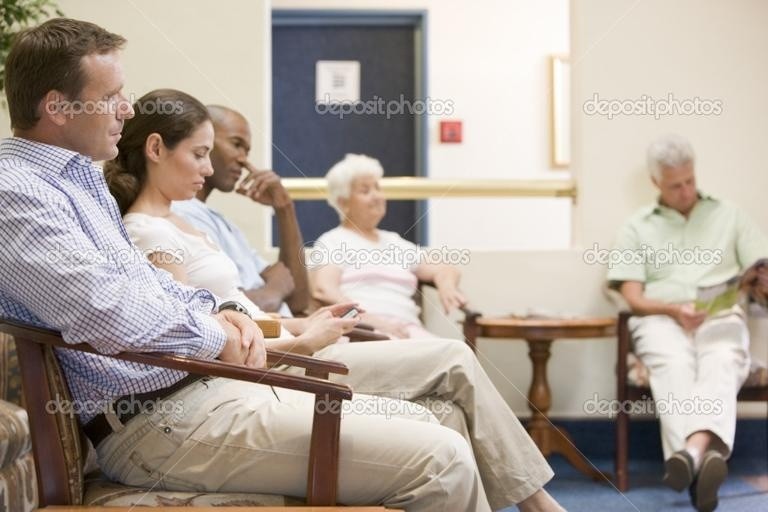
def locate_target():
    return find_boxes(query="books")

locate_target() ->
[694,258,768,318]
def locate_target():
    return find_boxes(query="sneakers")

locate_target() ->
[687,451,729,511]
[663,449,695,493]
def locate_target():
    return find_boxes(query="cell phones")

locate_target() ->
[341,308,358,319]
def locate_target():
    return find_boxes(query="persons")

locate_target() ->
[293,147,471,345]
[163,103,310,335]
[0,15,496,511]
[93,86,575,511]
[602,134,767,512]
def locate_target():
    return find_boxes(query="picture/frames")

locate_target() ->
[549,54,569,167]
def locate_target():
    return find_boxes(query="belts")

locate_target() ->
[84,374,205,447]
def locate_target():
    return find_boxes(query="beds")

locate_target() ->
[458,317,619,483]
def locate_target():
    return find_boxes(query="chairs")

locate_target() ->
[602,281,768,493]
[0,281,481,511]
